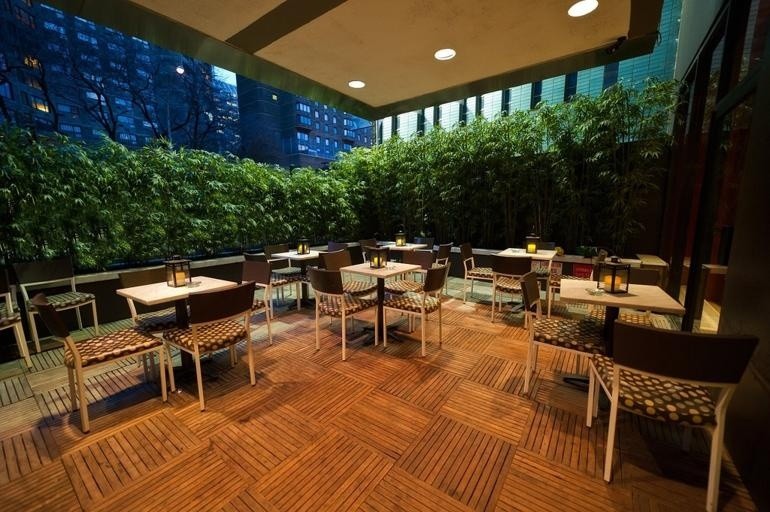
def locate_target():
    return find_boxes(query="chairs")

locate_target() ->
[118,265,178,376]
[0,252,35,369]
[163,278,257,412]
[31,292,169,435]
[12,255,100,353]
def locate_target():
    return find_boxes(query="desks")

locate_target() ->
[116,276,237,382]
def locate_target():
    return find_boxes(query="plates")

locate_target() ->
[385,266,396,269]
[185,282,202,288]
[585,288,605,295]
[511,250,520,252]
